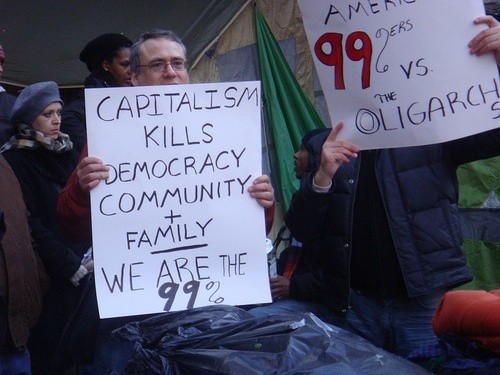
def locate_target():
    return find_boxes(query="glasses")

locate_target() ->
[134,59,188,73]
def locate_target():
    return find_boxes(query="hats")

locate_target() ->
[301,129,334,156]
[79,33,132,73]
[9,80,64,126]
[0,44,5,60]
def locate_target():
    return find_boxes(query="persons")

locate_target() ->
[0,154,42,375]
[59,33,134,154]
[270,127,334,301]
[0,81,82,375]
[283,15,500,358]
[56,27,277,243]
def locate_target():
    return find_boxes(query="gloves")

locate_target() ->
[69,265,88,286]
